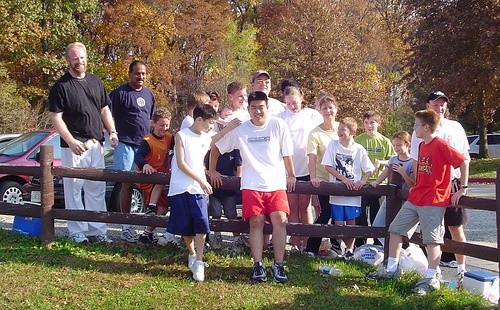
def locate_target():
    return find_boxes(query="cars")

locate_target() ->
[467,134,500,160]
[21,142,171,215]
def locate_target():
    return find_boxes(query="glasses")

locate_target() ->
[255,79,270,84]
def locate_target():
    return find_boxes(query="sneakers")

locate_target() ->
[412,279,440,296]
[138,230,162,248]
[188,252,198,270]
[120,228,137,243]
[253,261,268,282]
[271,259,288,284]
[439,259,458,269]
[71,232,89,243]
[164,228,364,260]
[365,267,400,282]
[90,234,113,243]
[191,261,205,281]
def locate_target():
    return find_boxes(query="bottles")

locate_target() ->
[318,266,343,276]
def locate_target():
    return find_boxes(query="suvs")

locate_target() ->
[0,129,114,207]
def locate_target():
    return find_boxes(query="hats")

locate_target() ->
[427,92,449,101]
[254,69,271,79]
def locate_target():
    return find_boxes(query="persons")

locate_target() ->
[135,107,176,244]
[371,130,418,262]
[305,91,356,261]
[179,69,331,145]
[353,110,398,253]
[320,117,375,261]
[208,90,297,284]
[275,83,325,257]
[409,90,472,290]
[166,105,242,283]
[437,106,460,270]
[202,145,247,257]
[106,59,157,244]
[374,109,469,296]
[46,41,119,246]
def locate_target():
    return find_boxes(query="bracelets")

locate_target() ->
[460,185,468,189]
[110,130,119,134]
